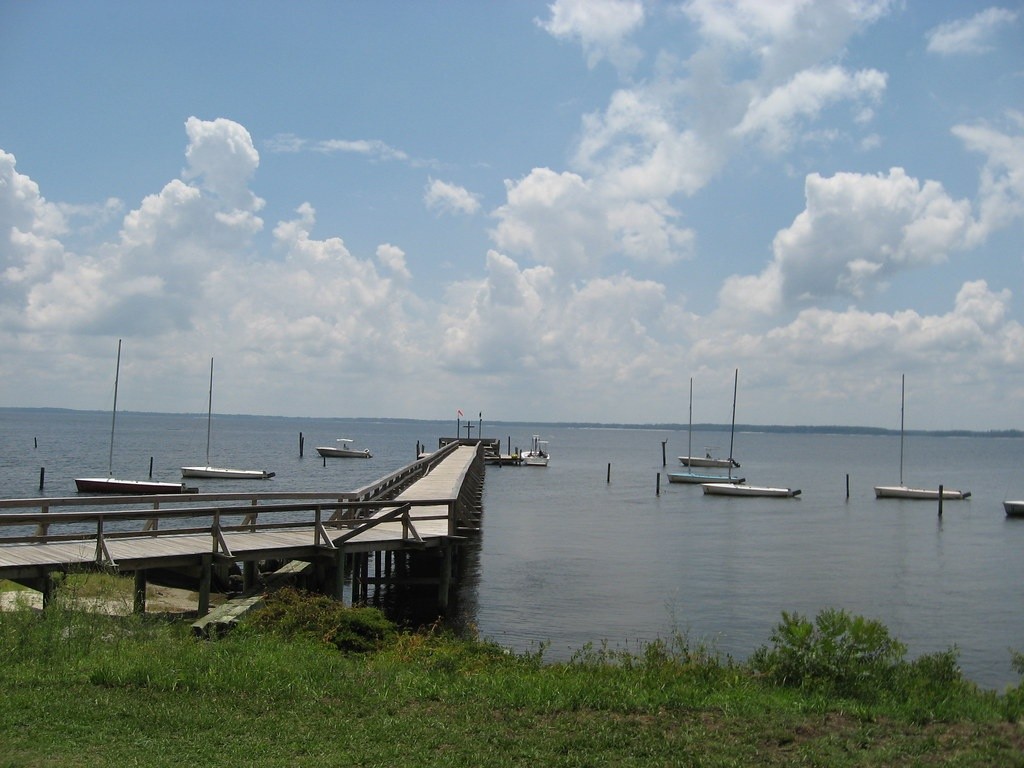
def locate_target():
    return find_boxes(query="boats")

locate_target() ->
[520,434,550,466]
[1002,500,1024,516]
[314,436,371,460]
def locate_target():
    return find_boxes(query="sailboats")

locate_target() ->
[678,377,741,468]
[701,369,803,497]
[180,356,276,479]
[873,374,973,501]
[73,338,183,495]
[666,377,745,485]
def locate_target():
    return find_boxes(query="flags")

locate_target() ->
[459,411,463,416]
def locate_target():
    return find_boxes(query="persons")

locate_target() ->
[528,453,533,457]
[343,444,349,450]
[539,450,544,457]
